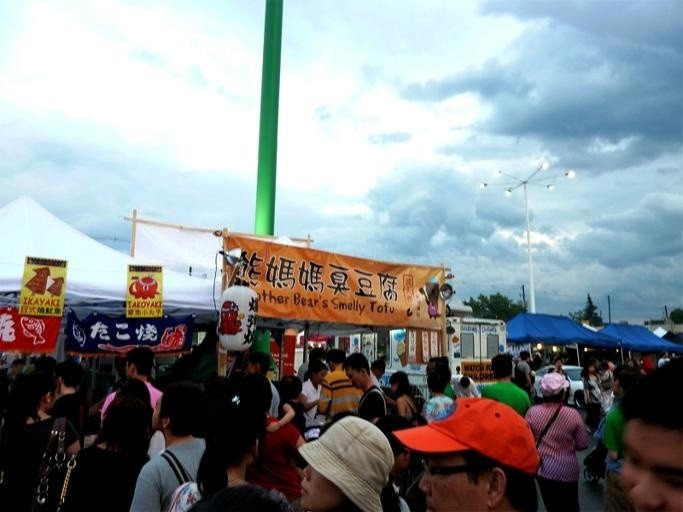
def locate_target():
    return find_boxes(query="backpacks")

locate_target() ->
[160,450,201,512]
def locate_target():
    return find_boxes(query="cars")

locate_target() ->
[531,365,587,411]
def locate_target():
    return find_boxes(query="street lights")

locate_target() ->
[477,161,576,315]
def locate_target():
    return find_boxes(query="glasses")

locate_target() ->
[421,457,475,476]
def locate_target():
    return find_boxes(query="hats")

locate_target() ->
[395,397,540,474]
[538,372,569,396]
[297,415,395,512]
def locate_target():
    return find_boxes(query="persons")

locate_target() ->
[2,338,682,512]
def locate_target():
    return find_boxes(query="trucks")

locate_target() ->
[334,318,507,400]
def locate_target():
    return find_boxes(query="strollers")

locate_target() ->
[583,440,605,483]
